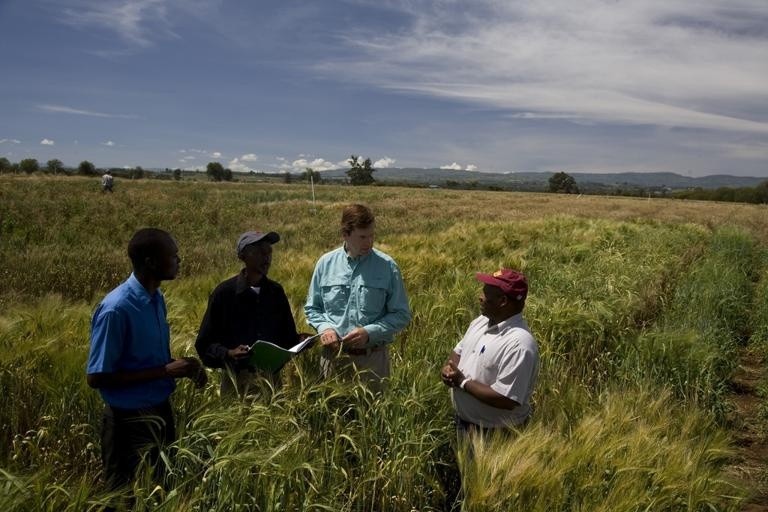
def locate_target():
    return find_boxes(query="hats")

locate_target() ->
[474,267,529,302]
[235,229,281,253]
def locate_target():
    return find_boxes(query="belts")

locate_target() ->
[346,346,383,355]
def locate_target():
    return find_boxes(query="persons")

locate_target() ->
[195,232,314,408]
[442,270,539,444]
[305,203,411,389]
[87,228,208,512]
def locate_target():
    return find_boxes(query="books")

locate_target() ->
[249,332,318,376]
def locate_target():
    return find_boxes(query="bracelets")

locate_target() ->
[460,378,472,392]
